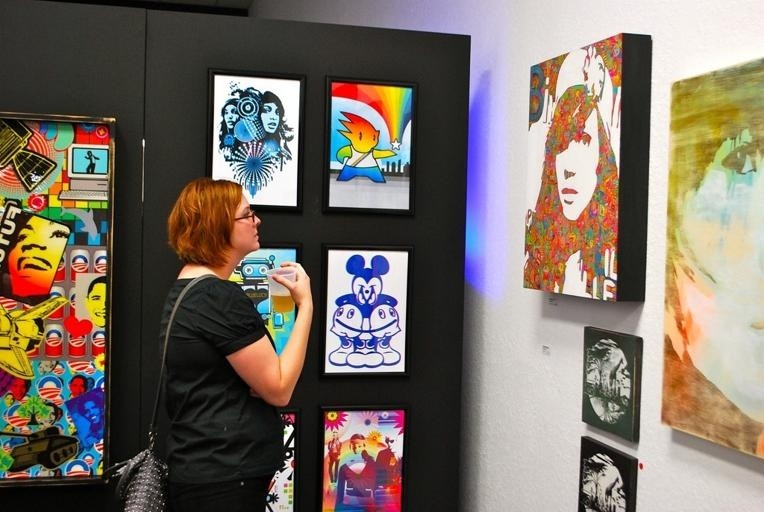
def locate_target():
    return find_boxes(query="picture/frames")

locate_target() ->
[230,241,414,380]
[578,325,642,509]
[266,403,410,512]
[206,66,419,221]
[1,111,120,490]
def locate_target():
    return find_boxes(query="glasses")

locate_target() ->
[235,210,256,222]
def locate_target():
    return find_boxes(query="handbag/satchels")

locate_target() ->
[102,449,169,511]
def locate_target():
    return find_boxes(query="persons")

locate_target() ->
[0,146,105,477]
[372,431,402,479]
[331,432,385,512]
[663,117,764,419]
[328,429,342,483]
[149,179,319,509]
[529,82,619,292]
[222,98,242,131]
[259,90,286,135]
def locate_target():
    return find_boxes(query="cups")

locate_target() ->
[266,267,297,313]
